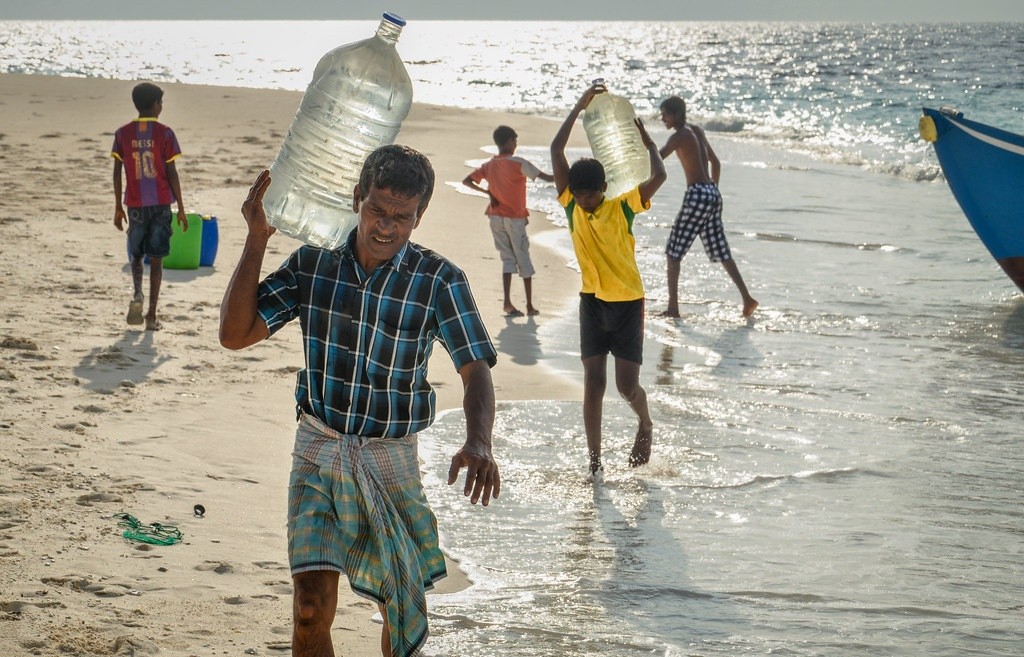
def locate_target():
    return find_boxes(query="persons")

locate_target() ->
[656,96,759,317]
[462,125,554,318]
[111,82,188,330]
[550,84,667,476]
[220,144,500,657]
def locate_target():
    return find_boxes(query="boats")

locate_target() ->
[919,106,1024,293]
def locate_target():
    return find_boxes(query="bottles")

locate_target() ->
[261,10,414,251]
[582,78,650,200]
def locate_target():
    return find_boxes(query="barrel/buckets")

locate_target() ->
[126,208,219,270]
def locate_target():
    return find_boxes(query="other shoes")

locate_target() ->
[145,319,163,330]
[126,301,143,325]
